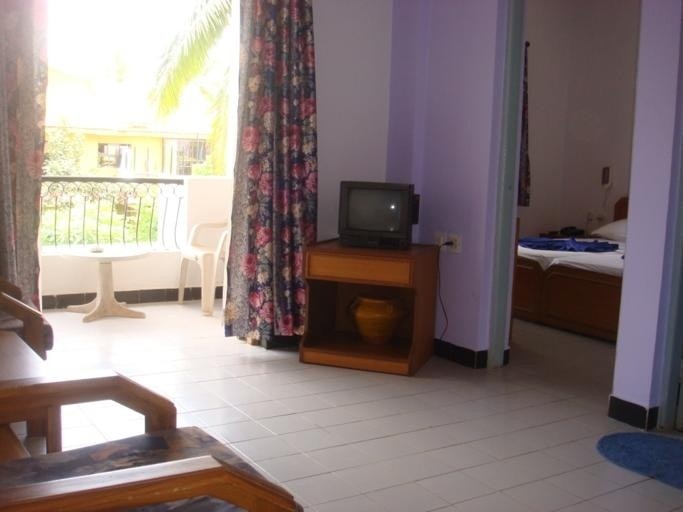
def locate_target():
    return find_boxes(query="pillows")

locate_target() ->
[588,218,627,242]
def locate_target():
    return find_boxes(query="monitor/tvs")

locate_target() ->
[339,181,414,250]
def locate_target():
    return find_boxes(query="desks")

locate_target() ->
[298,238,440,376]
[62,242,156,323]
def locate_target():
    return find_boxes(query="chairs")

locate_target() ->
[178,177,234,316]
[1,278,52,361]
[1,372,305,510]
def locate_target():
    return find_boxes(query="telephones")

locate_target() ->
[601,166,610,184]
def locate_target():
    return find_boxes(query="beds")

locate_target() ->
[514,196,629,343]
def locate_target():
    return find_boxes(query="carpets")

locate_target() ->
[597,429,682,490]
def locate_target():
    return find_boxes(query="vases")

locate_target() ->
[345,292,407,346]
